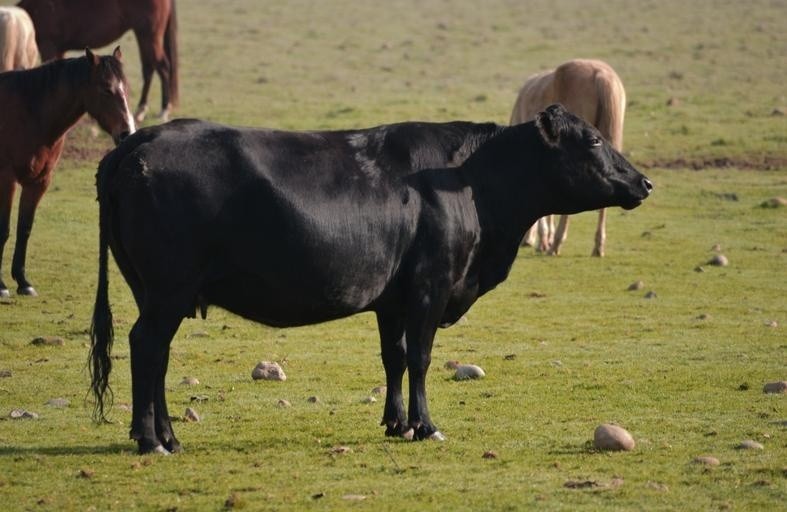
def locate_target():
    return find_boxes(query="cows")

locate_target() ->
[78,103,654,457]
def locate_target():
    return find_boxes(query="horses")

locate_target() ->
[15,0,180,122]
[509,57,626,258]
[0,45,138,295]
[0,5,39,73]
